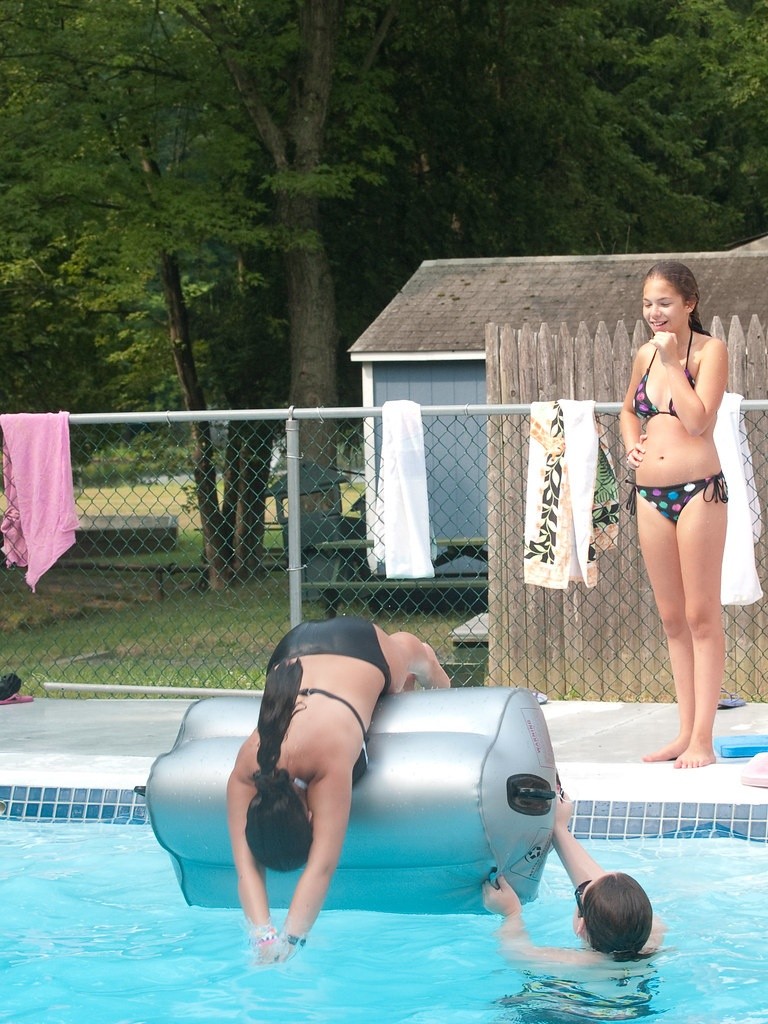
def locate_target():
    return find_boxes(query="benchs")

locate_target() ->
[352,572,487,578]
[449,613,489,677]
[301,580,488,589]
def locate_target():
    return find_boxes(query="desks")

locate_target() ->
[316,537,489,620]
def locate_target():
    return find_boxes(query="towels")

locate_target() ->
[0,410,82,594]
[713,390,768,607]
[372,399,438,580]
[523,398,620,590]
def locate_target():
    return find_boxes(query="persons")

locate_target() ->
[620,261,729,769]
[482,785,668,963]
[227,616,449,963]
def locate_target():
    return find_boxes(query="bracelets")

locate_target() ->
[627,449,634,459]
[254,929,306,947]
